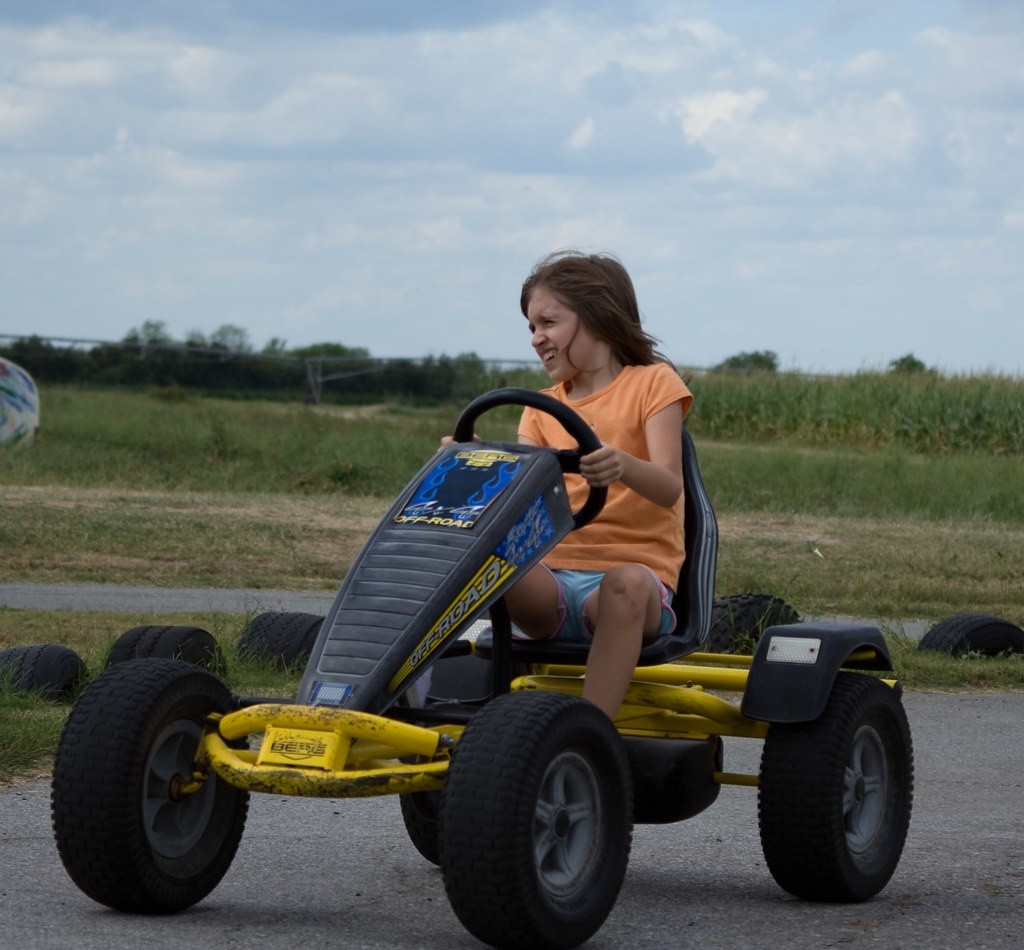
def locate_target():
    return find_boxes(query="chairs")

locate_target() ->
[502,423,719,670]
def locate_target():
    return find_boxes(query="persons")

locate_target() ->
[402,248,693,727]
